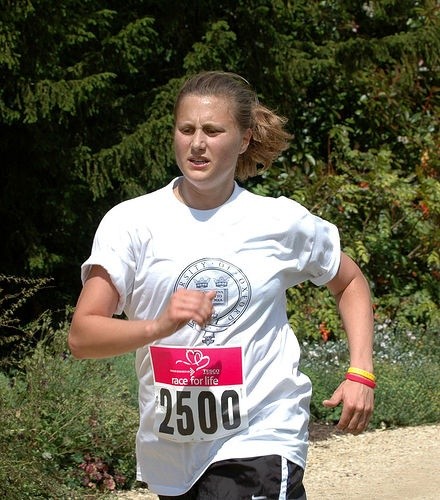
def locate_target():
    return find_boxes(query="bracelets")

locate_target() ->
[345,364,377,383]
[344,371,377,390]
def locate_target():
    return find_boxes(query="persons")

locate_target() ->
[62,71,380,500]
